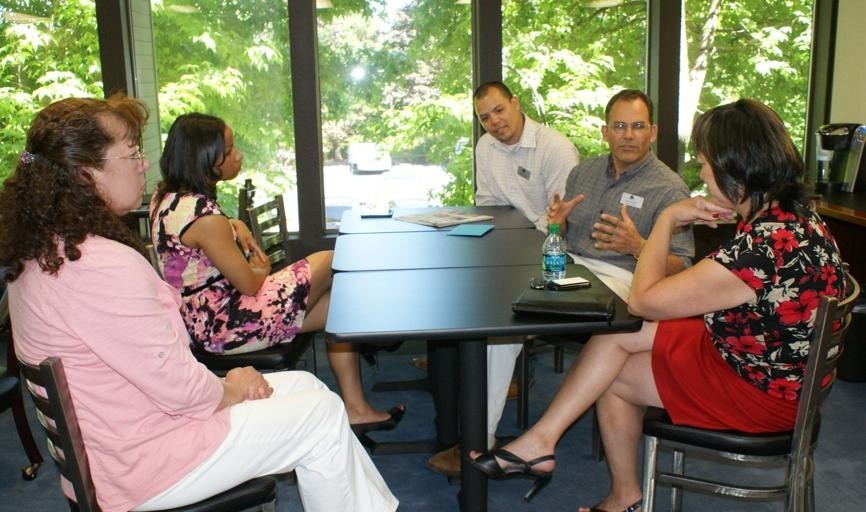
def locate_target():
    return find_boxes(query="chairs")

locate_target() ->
[14,356,279,512]
[0,277,44,481]
[643,262,861,512]
[146,245,318,375]
[245,194,293,276]
[238,180,257,234]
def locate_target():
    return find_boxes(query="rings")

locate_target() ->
[611,234,616,244]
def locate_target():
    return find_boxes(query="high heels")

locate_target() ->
[464,446,556,504]
[350,405,407,450]
[577,500,648,512]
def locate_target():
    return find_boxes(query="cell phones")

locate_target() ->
[361,209,393,219]
[549,277,591,292]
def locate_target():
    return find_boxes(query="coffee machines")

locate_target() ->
[813,122,866,212]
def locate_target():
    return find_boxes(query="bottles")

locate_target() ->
[543,223,567,279]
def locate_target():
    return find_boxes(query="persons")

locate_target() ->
[469,100,846,512]
[430,91,694,471]
[149,115,407,443]
[0,92,398,512]
[474,81,580,240]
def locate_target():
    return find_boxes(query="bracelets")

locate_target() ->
[633,254,640,261]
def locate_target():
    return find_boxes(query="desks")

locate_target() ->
[325,205,644,512]
[814,199,866,383]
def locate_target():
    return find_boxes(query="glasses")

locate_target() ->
[609,120,651,131]
[100,145,146,167]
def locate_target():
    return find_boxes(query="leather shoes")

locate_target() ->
[506,377,535,401]
[427,443,464,479]
